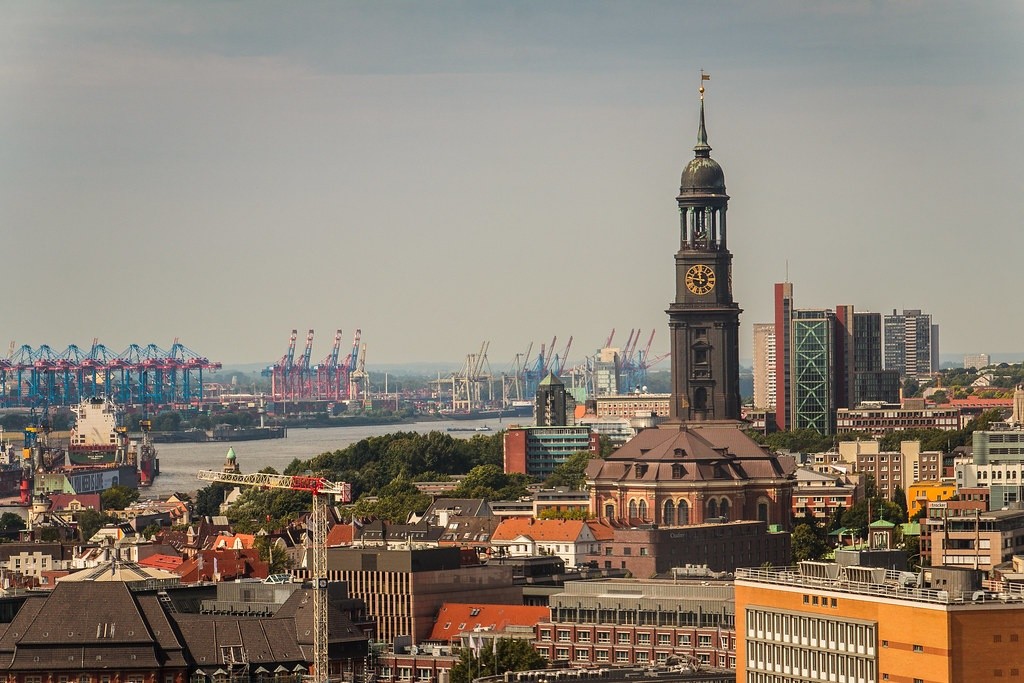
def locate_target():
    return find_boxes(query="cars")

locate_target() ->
[981,586,1021,602]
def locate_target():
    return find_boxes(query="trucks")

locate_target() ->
[897,571,916,588]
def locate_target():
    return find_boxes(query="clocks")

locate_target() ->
[685,264,716,295]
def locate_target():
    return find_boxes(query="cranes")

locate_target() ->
[196,469,345,682]
[263,326,671,406]
